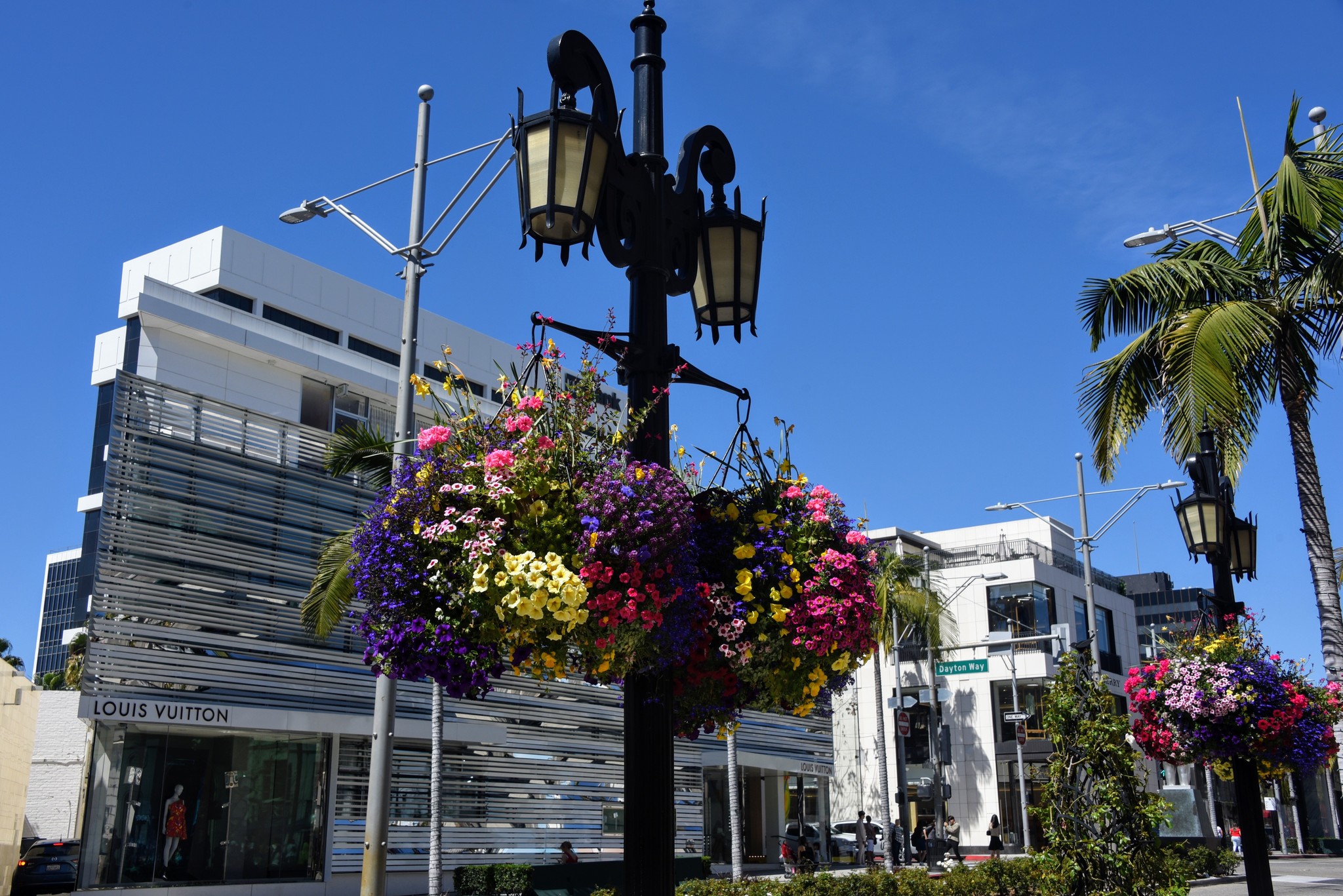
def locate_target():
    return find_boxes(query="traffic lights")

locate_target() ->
[939,724,953,764]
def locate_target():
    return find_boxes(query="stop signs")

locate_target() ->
[897,711,910,737]
[1016,724,1027,746]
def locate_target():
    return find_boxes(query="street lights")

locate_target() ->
[278,85,524,896]
[1167,403,1276,896]
[506,0,768,896]
[985,452,1186,690]
[916,546,1009,840]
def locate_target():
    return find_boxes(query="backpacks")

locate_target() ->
[911,828,924,847]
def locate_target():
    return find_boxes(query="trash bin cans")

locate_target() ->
[926,838,947,872]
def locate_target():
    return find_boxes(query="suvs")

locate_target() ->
[830,819,922,863]
[786,822,868,860]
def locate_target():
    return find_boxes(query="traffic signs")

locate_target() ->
[935,659,990,676]
[1004,711,1031,722]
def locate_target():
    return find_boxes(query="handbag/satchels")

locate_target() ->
[986,830,991,835]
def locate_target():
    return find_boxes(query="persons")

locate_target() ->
[944,815,963,865]
[865,815,876,867]
[923,819,937,868]
[1229,823,1242,855]
[557,840,578,864]
[797,836,815,874]
[161,784,187,881]
[914,820,926,866]
[1217,826,1223,840]
[855,810,867,868]
[889,818,904,866]
[810,842,820,875]
[988,814,1005,860]
